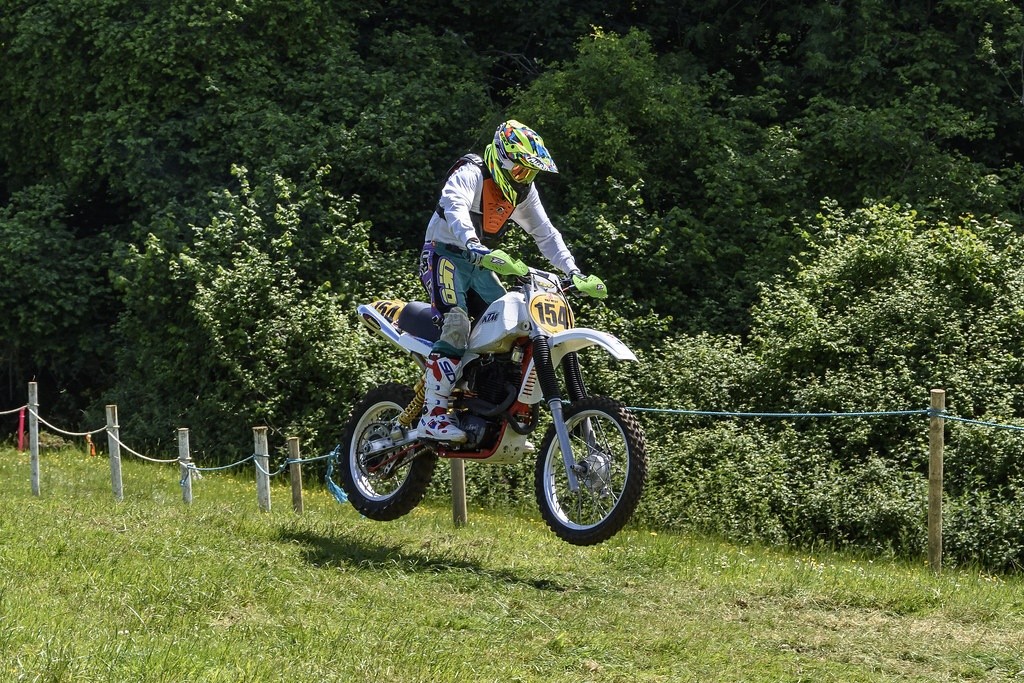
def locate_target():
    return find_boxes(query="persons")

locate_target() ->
[417,120,607,443]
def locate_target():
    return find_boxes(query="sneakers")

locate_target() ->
[523,439,535,453]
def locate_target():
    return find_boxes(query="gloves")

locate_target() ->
[570,270,589,297]
[466,239,491,267]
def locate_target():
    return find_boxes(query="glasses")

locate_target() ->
[496,132,540,184]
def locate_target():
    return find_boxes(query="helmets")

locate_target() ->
[484,119,559,207]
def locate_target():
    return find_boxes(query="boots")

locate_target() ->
[417,352,468,443]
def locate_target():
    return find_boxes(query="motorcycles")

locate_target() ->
[339,249,651,547]
